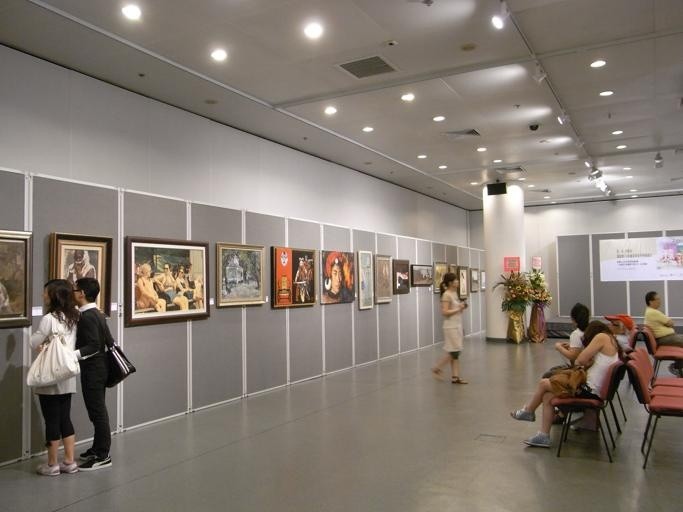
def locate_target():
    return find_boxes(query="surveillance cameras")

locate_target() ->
[528,121,539,131]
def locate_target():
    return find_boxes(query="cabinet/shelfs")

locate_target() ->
[529,302,549,344]
[505,306,526,346]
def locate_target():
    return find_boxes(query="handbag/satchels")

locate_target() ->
[26,315,80,386]
[105,346,135,387]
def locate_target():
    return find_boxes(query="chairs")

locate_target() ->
[554,315,683,470]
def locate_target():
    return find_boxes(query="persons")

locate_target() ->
[510,320,623,447]
[70,278,114,471]
[430,273,468,384]
[137,265,204,312]
[323,252,353,302]
[542,303,591,424]
[29,278,80,475]
[295,256,312,302]
[644,291,683,377]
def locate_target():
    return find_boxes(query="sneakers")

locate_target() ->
[569,416,600,431]
[78,449,100,460]
[510,409,535,422]
[35,462,60,475]
[552,409,565,424]
[78,454,111,470]
[58,461,78,473]
[521,435,552,447]
[668,364,682,378]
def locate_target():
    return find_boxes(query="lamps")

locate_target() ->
[653,150,665,171]
[491,0,509,30]
[556,109,571,127]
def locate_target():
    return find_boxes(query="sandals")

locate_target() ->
[451,375,467,384]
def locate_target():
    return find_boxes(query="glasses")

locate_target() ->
[610,320,618,326]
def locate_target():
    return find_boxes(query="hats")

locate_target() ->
[605,314,633,330]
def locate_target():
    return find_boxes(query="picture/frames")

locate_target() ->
[319,250,356,305]
[216,242,266,307]
[0,229,32,330]
[410,260,487,301]
[50,231,114,320]
[357,250,374,310]
[270,246,317,308]
[374,254,394,305]
[391,259,411,295]
[123,235,210,329]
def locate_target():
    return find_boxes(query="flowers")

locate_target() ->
[492,269,532,314]
[524,266,552,305]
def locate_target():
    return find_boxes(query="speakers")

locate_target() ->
[486,183,507,195]
